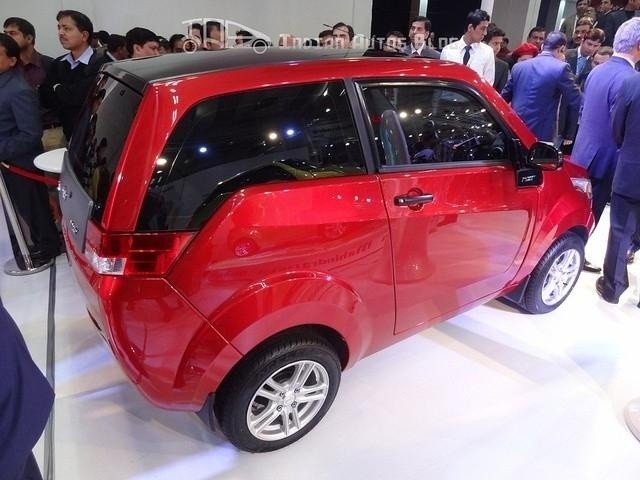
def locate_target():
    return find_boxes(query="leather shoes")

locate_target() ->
[27,245,61,268]
[595,277,625,304]
[625,251,635,261]
[581,259,601,274]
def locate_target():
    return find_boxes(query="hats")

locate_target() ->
[512,41,539,57]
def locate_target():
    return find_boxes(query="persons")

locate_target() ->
[569,15,640,273]
[2,14,57,132]
[0,295,56,480]
[596,70,640,311]
[50,0,640,165]
[0,32,67,263]
[72,88,112,211]
[412,116,503,163]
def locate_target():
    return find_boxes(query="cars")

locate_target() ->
[307,120,376,161]
[184,158,361,233]
[57,46,598,454]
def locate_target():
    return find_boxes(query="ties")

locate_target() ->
[463,46,471,65]
[577,56,588,77]
